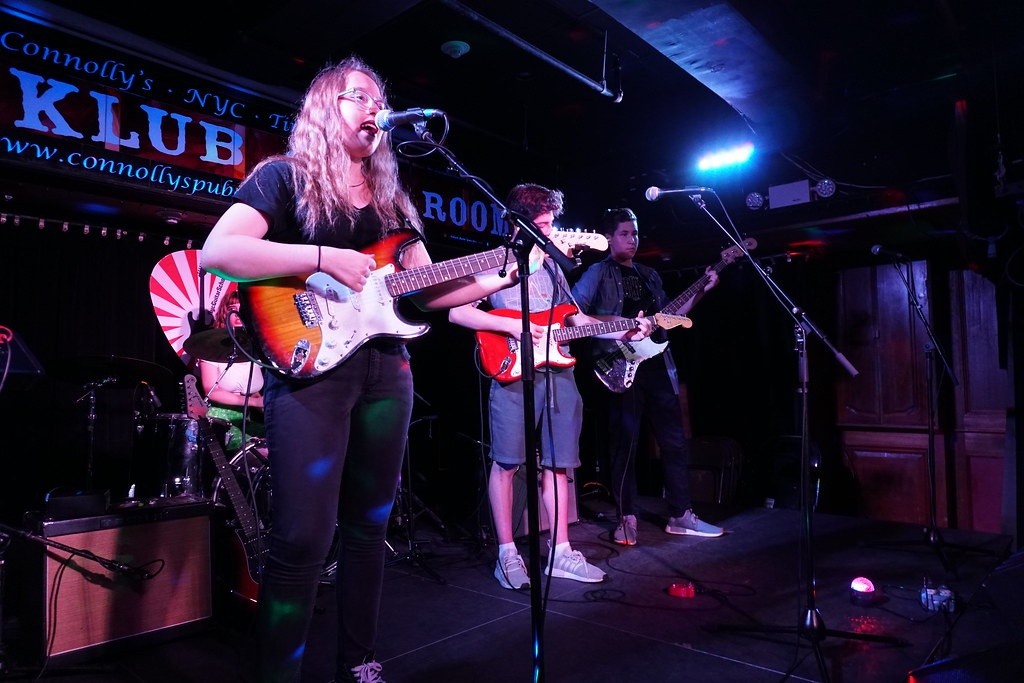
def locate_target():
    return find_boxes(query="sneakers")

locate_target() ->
[664,508,724,537]
[494,553,531,589]
[341,658,386,683]
[544,539,609,582]
[613,514,637,545]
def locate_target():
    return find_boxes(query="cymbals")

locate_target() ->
[182,324,261,364]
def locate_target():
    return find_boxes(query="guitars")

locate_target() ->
[181,373,269,608]
[588,239,758,394]
[474,304,693,383]
[237,227,608,381]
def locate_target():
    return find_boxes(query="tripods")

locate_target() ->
[685,192,1013,683]
[384,393,500,585]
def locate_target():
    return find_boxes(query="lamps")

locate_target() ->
[745,178,836,211]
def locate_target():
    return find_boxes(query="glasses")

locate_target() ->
[338,89,387,112]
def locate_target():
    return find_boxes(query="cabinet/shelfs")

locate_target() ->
[826,259,954,527]
[948,269,1014,535]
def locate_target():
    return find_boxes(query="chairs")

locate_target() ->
[669,433,825,512]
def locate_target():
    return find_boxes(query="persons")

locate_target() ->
[572,209,726,544]
[448,182,654,590]
[198,290,265,438]
[197,60,550,682]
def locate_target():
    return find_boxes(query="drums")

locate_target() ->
[217,429,272,545]
[139,410,207,506]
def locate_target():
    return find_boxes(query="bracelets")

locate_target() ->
[317,244,321,270]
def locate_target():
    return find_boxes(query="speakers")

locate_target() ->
[23,499,215,666]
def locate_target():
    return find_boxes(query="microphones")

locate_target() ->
[375,107,447,132]
[871,245,910,260]
[128,563,153,579]
[644,186,714,202]
[145,383,162,408]
